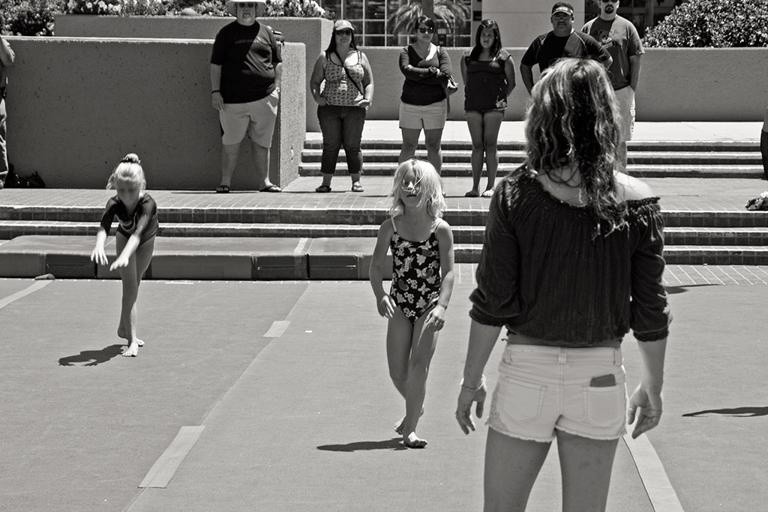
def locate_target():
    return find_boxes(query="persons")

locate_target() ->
[0,34,16,188]
[519,1,614,96]
[580,0,646,174]
[368,157,455,447]
[207,0,283,194]
[309,19,374,192]
[87,151,160,359]
[397,14,452,176]
[454,57,673,512]
[459,17,518,198]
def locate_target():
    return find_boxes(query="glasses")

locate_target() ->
[418,27,434,34]
[239,2,254,8]
[336,29,351,36]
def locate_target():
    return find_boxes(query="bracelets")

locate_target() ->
[459,373,486,391]
[211,89,221,95]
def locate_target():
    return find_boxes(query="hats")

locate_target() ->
[226,0,266,17]
[333,19,355,31]
[551,1,574,18]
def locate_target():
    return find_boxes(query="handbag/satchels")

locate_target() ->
[441,75,458,96]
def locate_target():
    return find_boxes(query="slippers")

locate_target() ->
[464,189,480,196]
[259,185,282,193]
[316,185,331,192]
[352,185,364,192]
[216,184,230,193]
[481,187,494,198]
